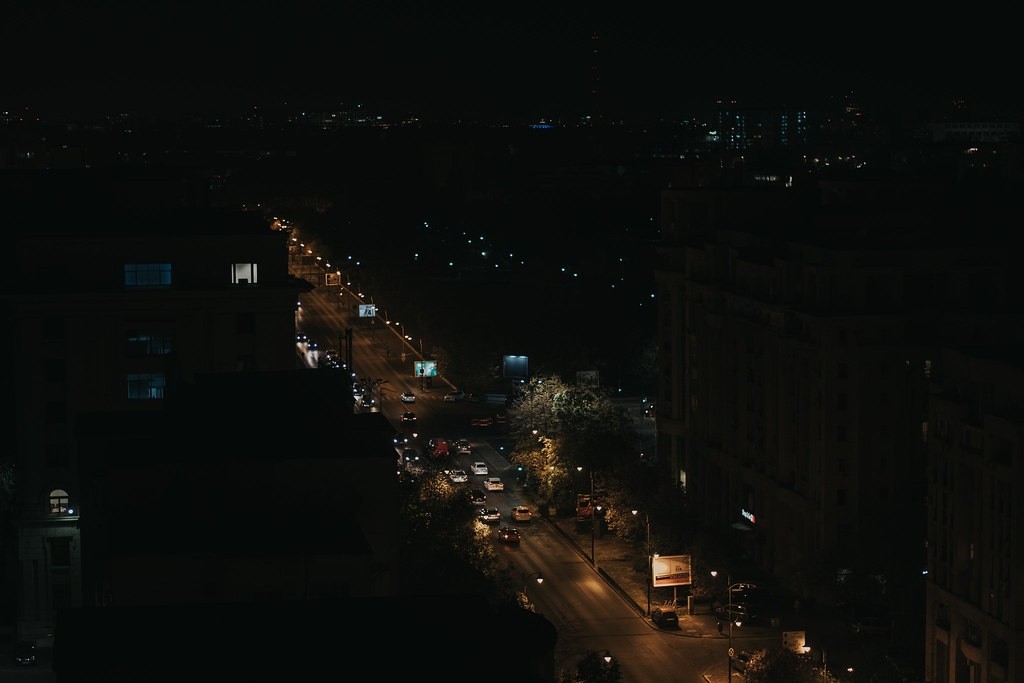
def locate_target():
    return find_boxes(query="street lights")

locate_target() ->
[577,466,595,565]
[727,583,758,683]
[278,221,378,342]
[630,507,653,618]
[386,320,405,352]
[405,334,424,394]
[532,428,547,486]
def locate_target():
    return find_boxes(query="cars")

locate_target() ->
[652,605,678,628]
[395,430,471,485]
[401,391,414,403]
[296,331,308,343]
[444,390,466,402]
[324,344,376,408]
[512,505,532,522]
[484,477,504,491]
[715,582,758,626]
[498,526,521,544]
[468,490,487,507]
[400,412,417,425]
[306,338,319,350]
[480,506,501,524]
[471,461,489,475]
